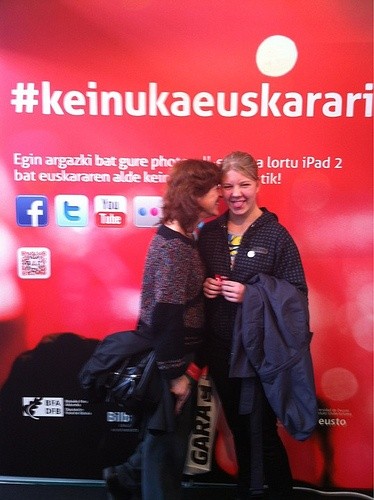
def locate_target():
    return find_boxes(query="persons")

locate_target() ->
[128,159,223,500]
[193,153,314,498]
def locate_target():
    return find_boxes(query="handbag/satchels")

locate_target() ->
[80,329,161,416]
